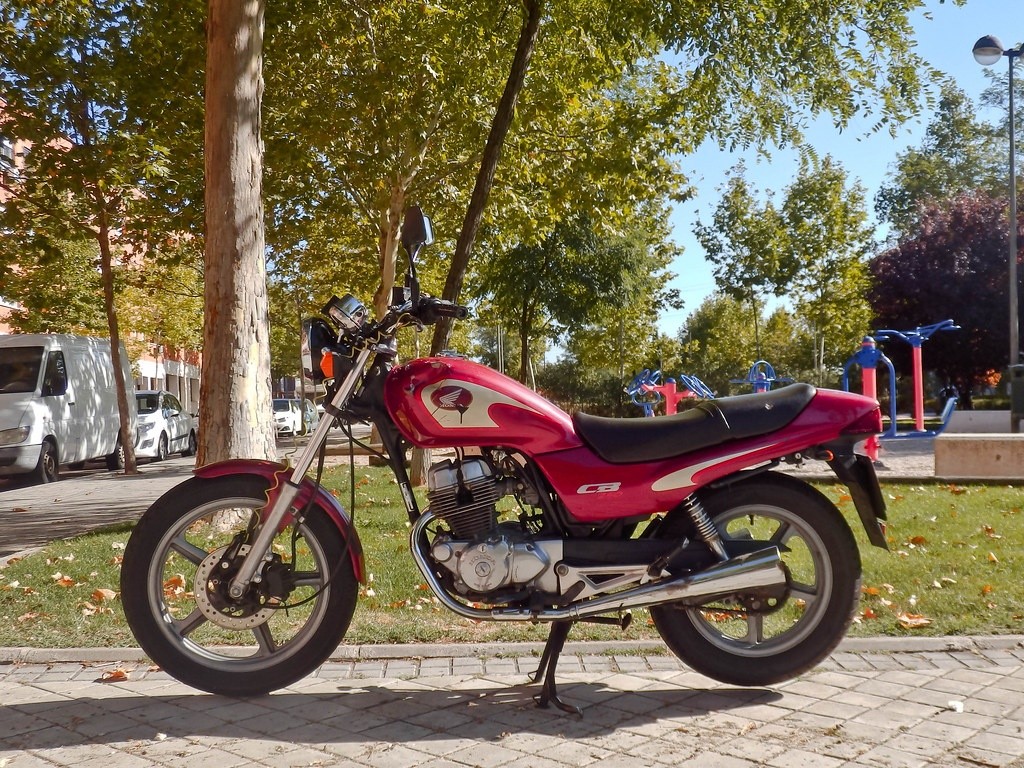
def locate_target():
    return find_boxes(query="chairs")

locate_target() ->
[147,397,156,410]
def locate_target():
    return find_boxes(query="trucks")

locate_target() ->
[0,332,134,484]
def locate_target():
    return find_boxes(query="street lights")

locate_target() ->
[971,35,1023,414]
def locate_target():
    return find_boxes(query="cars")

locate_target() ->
[124,390,199,460]
[274,396,319,437]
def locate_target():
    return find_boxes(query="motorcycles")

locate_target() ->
[120,206,886,714]
